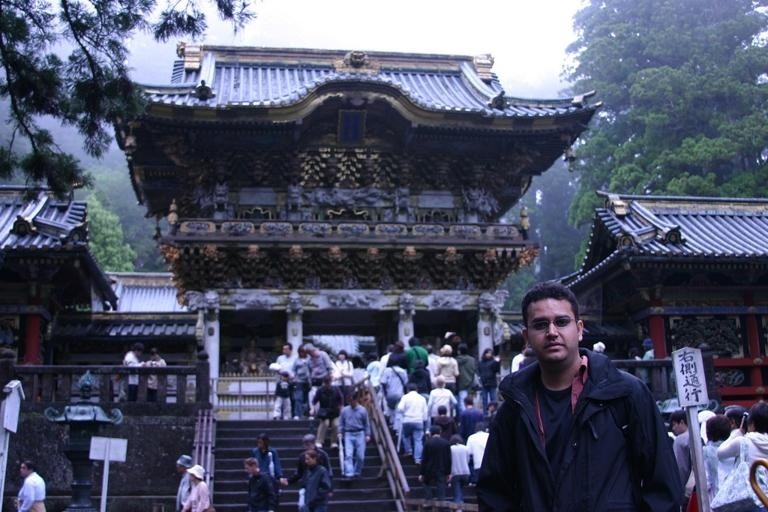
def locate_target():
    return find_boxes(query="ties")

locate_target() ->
[346,428,361,432]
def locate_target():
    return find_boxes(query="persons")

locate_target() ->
[273,372,292,421]
[330,361,343,389]
[302,344,333,416]
[123,343,144,401]
[335,350,353,387]
[242,457,275,512]
[174,455,192,512]
[367,330,500,512]
[293,344,315,420]
[146,348,167,402]
[182,464,210,512]
[312,376,344,449]
[511,343,534,374]
[297,450,331,512]
[279,434,333,504]
[479,284,680,512]
[627,337,655,387]
[18,460,48,512]
[671,401,767,512]
[251,433,282,480]
[274,343,298,379]
[338,393,370,479]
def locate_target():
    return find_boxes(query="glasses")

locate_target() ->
[533,318,570,330]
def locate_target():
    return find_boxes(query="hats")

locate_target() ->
[176,455,192,468]
[186,465,204,480]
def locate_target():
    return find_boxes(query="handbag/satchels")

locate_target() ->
[710,460,768,512]
[317,407,334,419]
[311,378,323,386]
